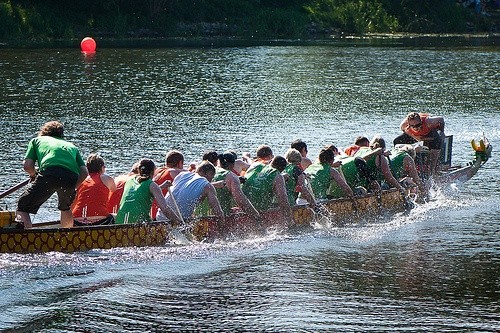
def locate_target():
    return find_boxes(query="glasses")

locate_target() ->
[410,123,421,127]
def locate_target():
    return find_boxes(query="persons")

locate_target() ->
[70,134,431,246]
[17,120,89,229]
[393,112,445,178]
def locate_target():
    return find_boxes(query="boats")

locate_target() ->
[0,137,494,255]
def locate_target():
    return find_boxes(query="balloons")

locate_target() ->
[80,36,96,50]
